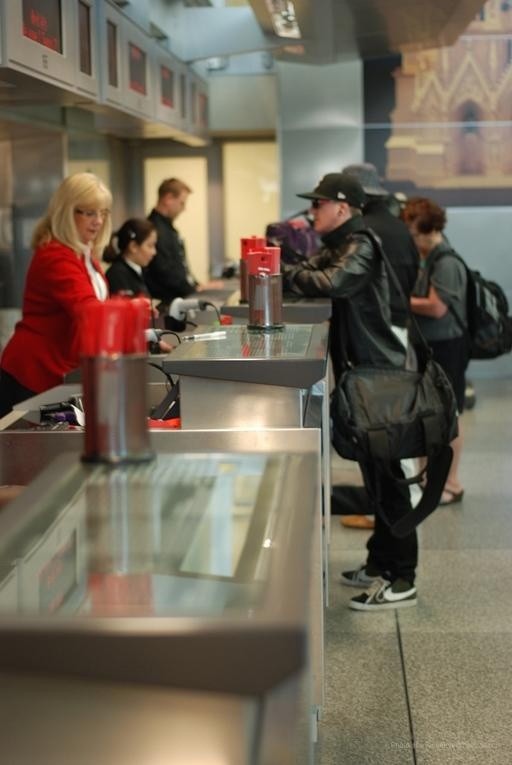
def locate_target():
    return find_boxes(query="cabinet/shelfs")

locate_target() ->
[1,2,214,154]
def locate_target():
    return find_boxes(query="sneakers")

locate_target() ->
[338,567,394,590]
[340,514,378,530]
[347,576,419,612]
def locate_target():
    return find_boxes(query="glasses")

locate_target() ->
[311,201,324,208]
[74,206,110,219]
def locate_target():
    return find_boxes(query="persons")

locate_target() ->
[0,170,173,420]
[102,217,172,353]
[142,177,199,305]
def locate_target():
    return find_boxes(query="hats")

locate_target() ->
[295,171,368,210]
[338,161,389,197]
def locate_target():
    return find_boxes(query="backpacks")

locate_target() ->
[421,248,512,360]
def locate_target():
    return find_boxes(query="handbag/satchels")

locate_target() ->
[325,359,461,539]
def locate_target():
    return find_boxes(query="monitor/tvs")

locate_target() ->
[222,286,332,323]
[163,322,329,388]
[0,451,318,694]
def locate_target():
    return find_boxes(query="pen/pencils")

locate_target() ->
[180,331,229,341]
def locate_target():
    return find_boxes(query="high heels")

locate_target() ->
[435,487,466,507]
